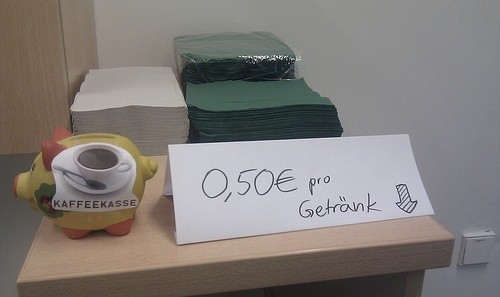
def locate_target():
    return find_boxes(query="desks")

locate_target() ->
[14,152,454,297]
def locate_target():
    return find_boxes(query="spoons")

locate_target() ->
[53,165,106,189]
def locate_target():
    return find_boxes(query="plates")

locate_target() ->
[62,158,132,194]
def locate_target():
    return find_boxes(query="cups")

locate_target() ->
[73,147,132,179]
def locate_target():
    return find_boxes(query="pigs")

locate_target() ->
[13,126,158,239]
